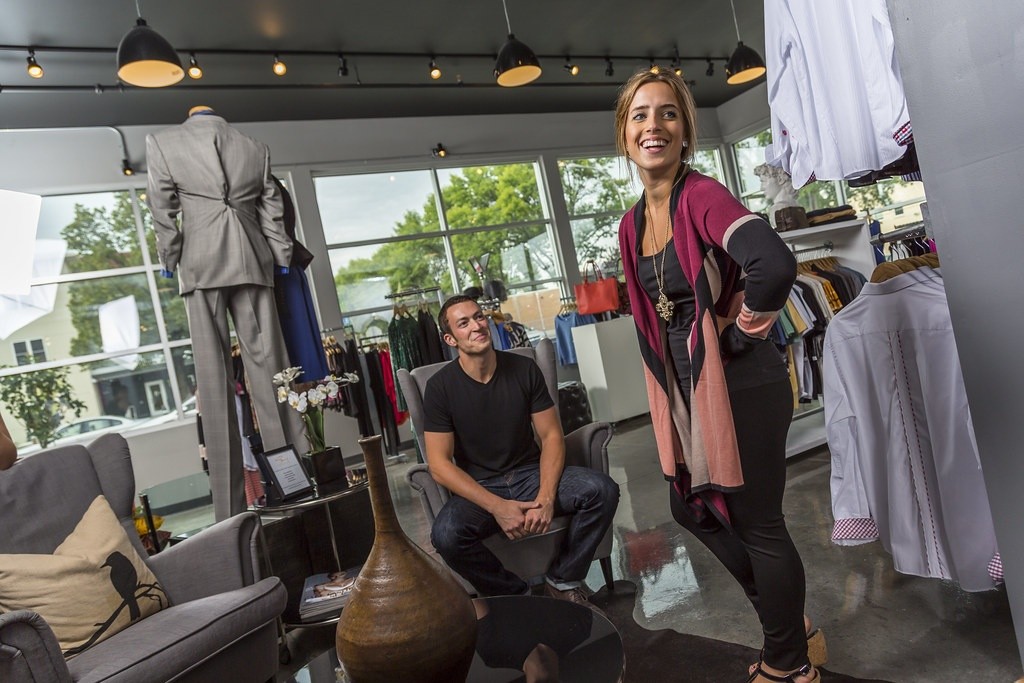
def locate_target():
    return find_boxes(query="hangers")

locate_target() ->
[793,225,942,282]
[479,301,505,325]
[231,343,241,357]
[321,325,389,357]
[557,296,577,315]
[393,287,428,317]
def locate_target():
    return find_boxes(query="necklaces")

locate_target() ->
[645,188,675,321]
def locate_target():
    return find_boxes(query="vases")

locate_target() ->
[336,433,478,683]
[301,445,347,485]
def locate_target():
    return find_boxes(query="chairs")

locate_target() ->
[397,337,615,594]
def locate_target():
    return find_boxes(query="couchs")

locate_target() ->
[0,433,289,683]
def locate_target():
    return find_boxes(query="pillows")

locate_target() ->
[0,495,172,661]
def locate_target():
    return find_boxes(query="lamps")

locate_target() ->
[122,160,133,175]
[27,0,766,87]
[432,142,446,159]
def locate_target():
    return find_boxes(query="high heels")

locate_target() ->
[743,657,821,683]
[748,628,830,676]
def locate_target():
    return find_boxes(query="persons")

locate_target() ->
[110,384,134,418]
[424,295,621,618]
[314,571,360,597]
[464,600,594,683]
[146,105,313,524]
[0,413,17,470]
[617,68,828,683]
[463,277,512,333]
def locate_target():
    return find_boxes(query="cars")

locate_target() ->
[139,395,198,435]
[15,415,154,459]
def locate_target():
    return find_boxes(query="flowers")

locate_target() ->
[272,366,358,451]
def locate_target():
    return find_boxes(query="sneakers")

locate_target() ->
[544,583,607,618]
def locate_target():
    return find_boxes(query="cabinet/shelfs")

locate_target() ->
[169,514,312,638]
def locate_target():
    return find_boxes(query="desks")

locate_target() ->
[249,469,372,663]
[283,596,628,683]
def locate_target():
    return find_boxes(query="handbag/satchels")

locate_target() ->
[611,258,632,316]
[573,261,620,316]
